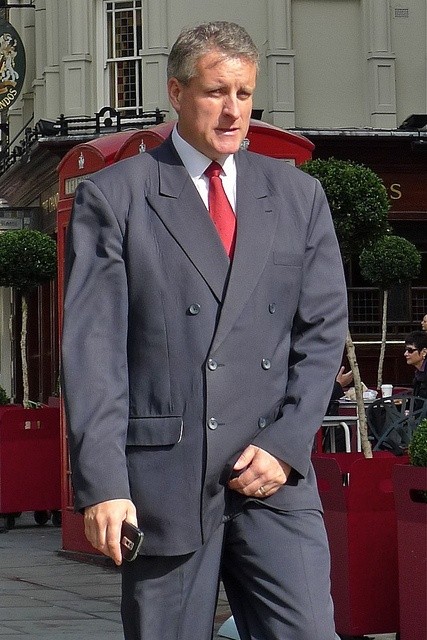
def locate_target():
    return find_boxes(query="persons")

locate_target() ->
[61,23,348,635]
[321,366,353,454]
[403,332,426,411]
[421,313,427,331]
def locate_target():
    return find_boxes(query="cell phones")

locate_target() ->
[119,521,145,562]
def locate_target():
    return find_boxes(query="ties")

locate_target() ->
[204,162,236,260]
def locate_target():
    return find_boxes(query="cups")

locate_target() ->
[380,384,392,399]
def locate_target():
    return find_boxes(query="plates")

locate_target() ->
[385,398,408,405]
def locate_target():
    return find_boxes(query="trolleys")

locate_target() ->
[0,402,62,527]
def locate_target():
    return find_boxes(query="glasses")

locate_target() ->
[405,346,422,354]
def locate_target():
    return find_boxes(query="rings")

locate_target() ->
[260,486,267,495]
